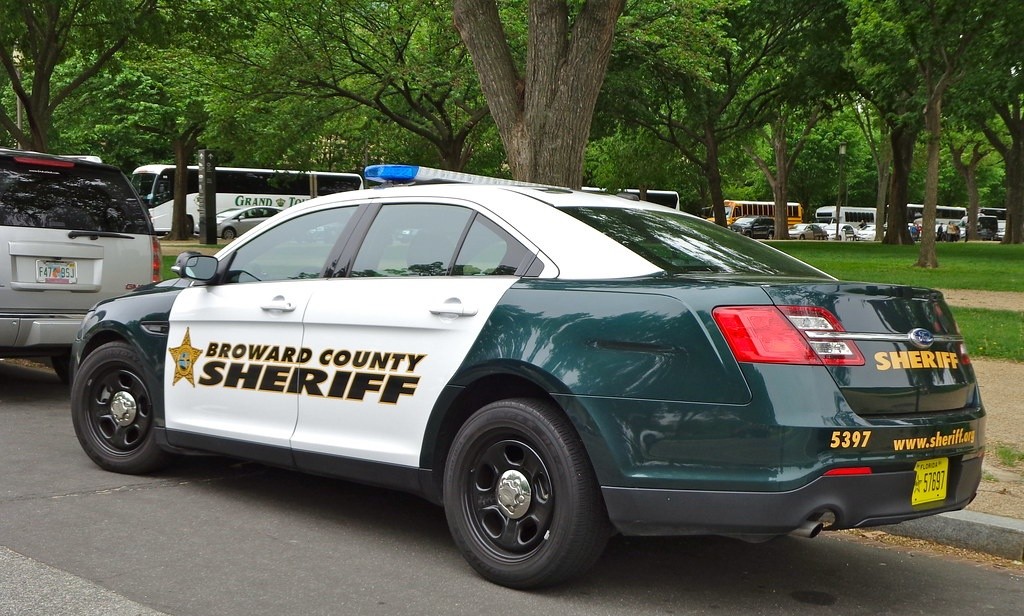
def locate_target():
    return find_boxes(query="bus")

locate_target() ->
[814,205,876,230]
[581,186,680,211]
[907,203,967,240]
[130,163,364,238]
[701,198,803,229]
[978,207,1006,241]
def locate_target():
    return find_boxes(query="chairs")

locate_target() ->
[405,228,467,274]
[255,211,264,216]
[351,228,385,275]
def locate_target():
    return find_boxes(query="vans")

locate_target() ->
[957,215,998,241]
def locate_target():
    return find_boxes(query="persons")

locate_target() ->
[912,224,920,241]
[935,221,958,243]
[856,219,866,229]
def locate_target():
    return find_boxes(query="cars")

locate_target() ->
[824,222,858,242]
[66,162,990,594]
[788,224,827,240]
[194,206,286,240]
[855,224,887,241]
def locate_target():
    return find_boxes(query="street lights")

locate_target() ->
[835,141,850,241]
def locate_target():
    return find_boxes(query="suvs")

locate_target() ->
[729,214,775,240]
[0,147,163,386]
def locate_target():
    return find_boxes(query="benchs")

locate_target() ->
[812,230,830,240]
[844,231,861,241]
